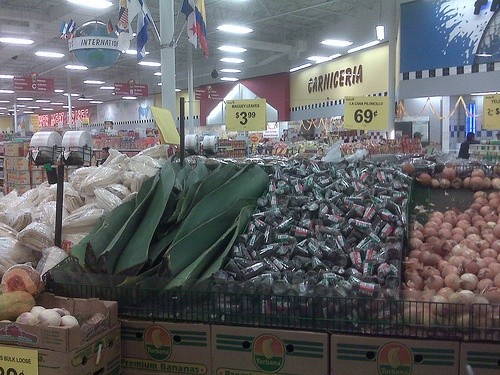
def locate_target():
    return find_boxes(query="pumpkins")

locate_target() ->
[0,265,43,321]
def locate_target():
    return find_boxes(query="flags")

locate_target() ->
[181,0,209,59]
[108,20,113,32]
[67,19,76,34]
[118,0,148,62]
[61,21,68,35]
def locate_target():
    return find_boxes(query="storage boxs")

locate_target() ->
[0,293,500,375]
[0,133,161,197]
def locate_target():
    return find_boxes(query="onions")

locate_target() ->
[400,168,500,328]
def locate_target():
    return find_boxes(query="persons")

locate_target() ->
[458,132,480,159]
[414,132,423,140]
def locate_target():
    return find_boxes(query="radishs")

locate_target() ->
[0,306,79,328]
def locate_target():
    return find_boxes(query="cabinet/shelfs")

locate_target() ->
[217,138,247,158]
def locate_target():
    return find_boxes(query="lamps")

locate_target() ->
[375,24,385,41]
